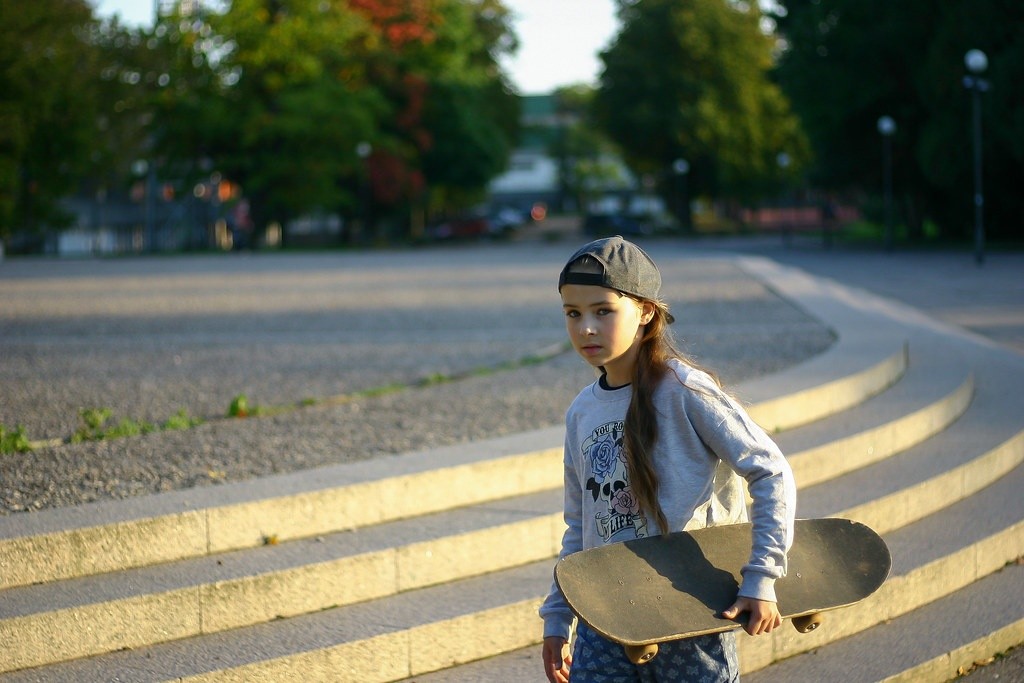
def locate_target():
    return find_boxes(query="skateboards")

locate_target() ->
[554,517,892,662]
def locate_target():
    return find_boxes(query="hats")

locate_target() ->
[558,235,674,323]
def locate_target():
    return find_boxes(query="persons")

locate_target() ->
[538,233,796,683]
